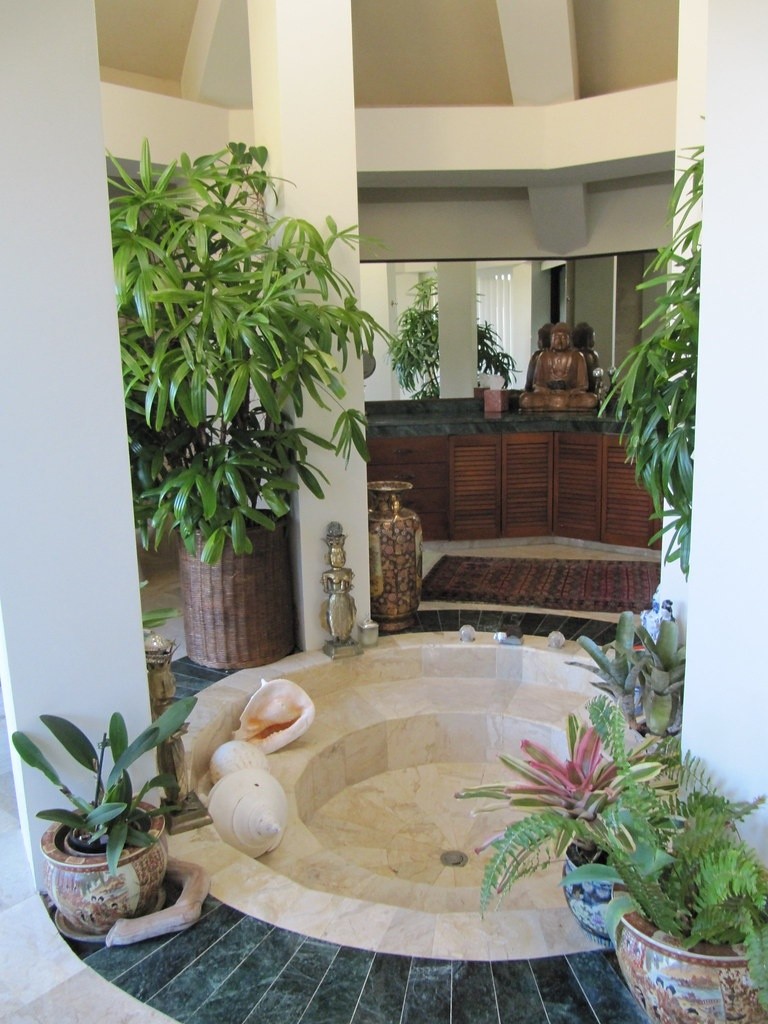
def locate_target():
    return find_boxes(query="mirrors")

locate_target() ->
[360,251,668,401]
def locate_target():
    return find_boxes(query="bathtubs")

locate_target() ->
[164,628,666,965]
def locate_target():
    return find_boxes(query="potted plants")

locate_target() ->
[616,790,729,1006]
[10,694,202,940]
[562,611,688,756]
[107,142,398,667]
[453,692,692,950]
[646,787,768,1024]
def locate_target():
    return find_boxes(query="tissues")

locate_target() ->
[473,371,490,400]
[483,374,510,413]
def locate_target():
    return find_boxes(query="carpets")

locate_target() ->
[418,555,660,615]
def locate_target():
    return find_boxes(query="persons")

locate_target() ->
[321,522,364,660]
[571,322,607,393]
[645,599,678,642]
[525,323,554,393]
[518,323,599,415]
